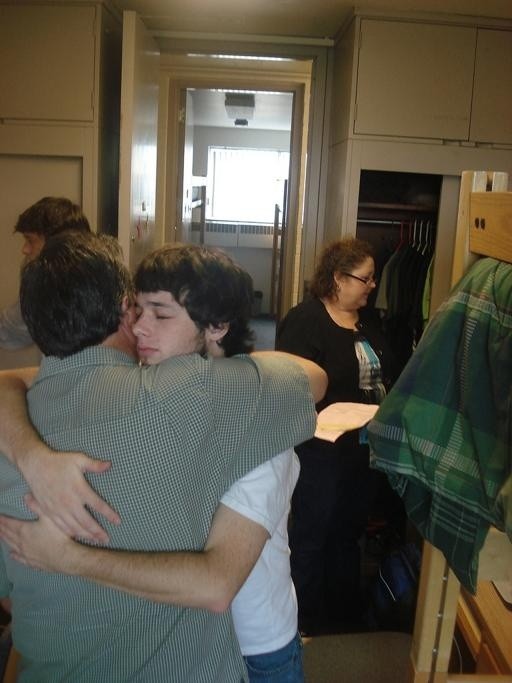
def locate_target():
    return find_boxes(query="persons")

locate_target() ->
[0,194,92,347]
[276,236,390,635]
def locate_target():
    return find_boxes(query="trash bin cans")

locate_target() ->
[251,291,262,316]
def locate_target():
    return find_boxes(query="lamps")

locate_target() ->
[224,93,255,128]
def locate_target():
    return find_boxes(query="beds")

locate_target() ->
[403,162,512,677]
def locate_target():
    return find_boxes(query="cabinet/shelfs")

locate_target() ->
[2,35,99,310]
[322,11,510,398]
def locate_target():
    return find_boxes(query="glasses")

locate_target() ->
[343,272,372,285]
